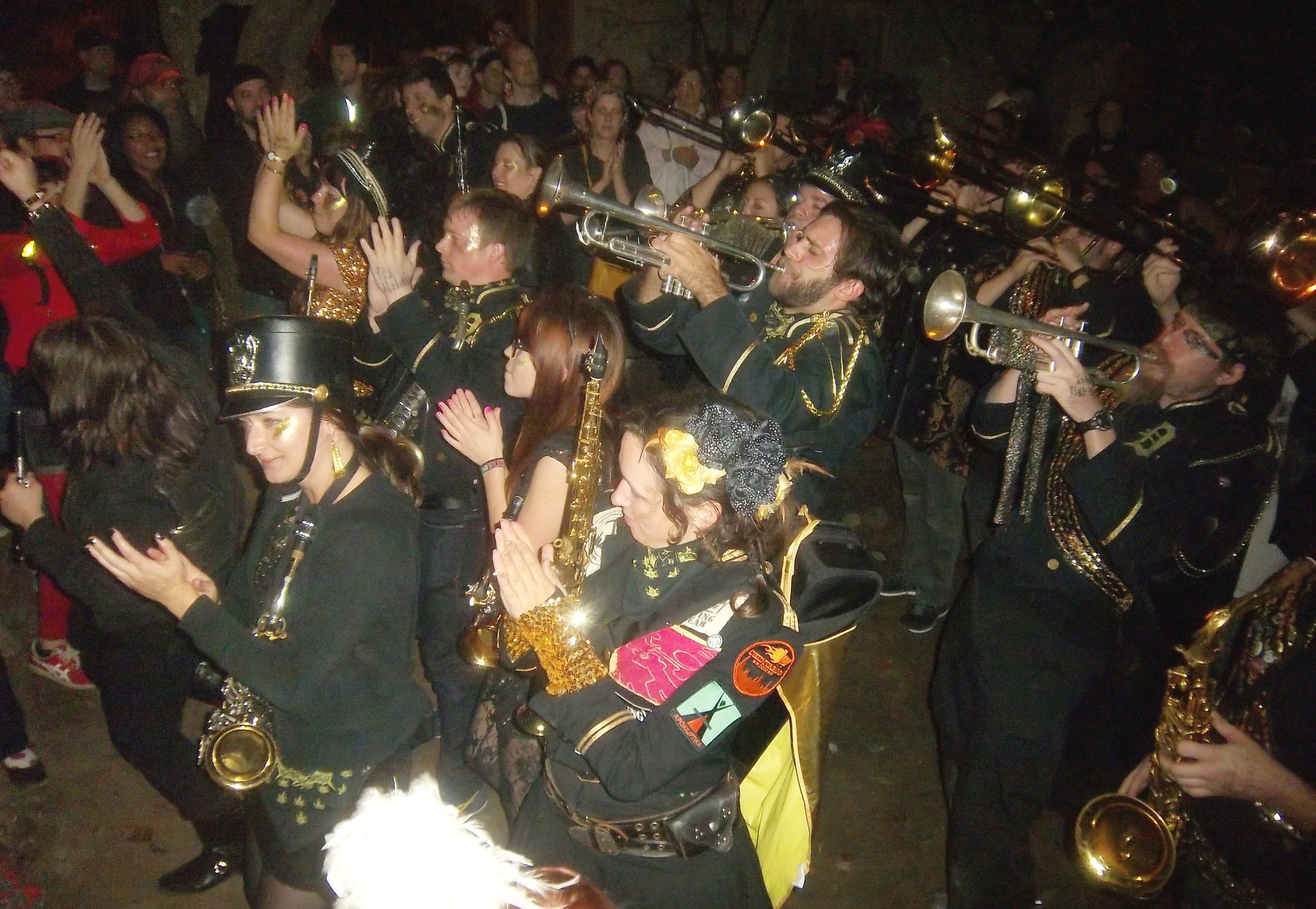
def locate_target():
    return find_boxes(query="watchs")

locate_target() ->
[1076,409,1116,433]
[264,150,289,164]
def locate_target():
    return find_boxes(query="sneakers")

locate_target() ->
[27,639,96,689]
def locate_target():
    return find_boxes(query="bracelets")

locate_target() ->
[480,458,506,474]
[261,162,286,174]
[1069,265,1089,281]
[22,186,46,208]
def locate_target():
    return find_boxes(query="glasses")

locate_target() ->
[1173,312,1221,361]
[125,130,162,141]
[32,131,66,142]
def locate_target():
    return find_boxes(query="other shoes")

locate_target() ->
[903,602,952,633]
[10,759,49,787]
[879,573,917,596]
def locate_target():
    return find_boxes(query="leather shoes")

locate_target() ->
[159,855,245,894]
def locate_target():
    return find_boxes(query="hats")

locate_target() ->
[129,54,179,90]
[333,139,388,222]
[804,144,870,210]
[219,315,354,421]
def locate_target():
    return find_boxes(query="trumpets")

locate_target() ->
[919,271,1158,391]
[534,153,787,300]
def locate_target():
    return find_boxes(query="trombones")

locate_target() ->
[908,117,1236,285]
[622,81,905,199]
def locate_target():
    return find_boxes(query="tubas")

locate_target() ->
[1243,212,1315,305]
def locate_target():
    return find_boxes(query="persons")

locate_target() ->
[0,12,1316,909]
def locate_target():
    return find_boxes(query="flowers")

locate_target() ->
[657,431,728,497]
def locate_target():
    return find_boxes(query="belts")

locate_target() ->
[533,758,743,861]
[420,495,470,510]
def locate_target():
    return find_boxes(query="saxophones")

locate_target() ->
[514,333,607,737]
[199,523,313,792]
[1069,549,1315,905]
[632,186,805,257]
[359,280,478,489]
